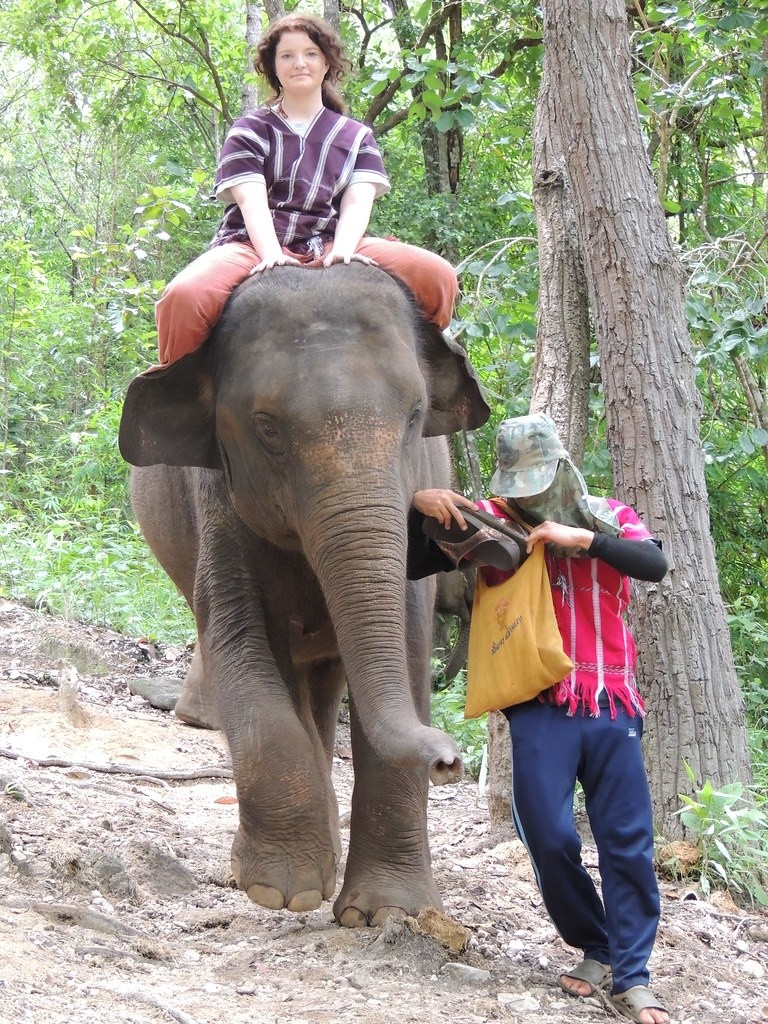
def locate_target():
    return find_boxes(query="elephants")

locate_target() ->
[114,260,489,927]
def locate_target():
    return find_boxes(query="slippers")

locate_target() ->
[421,506,534,573]
[607,986,671,1024]
[556,958,613,997]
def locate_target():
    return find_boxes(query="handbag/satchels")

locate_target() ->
[462,498,575,720]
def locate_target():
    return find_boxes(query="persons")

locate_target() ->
[155,11,458,368]
[406,412,671,1024]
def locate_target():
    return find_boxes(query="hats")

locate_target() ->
[487,411,625,535]
